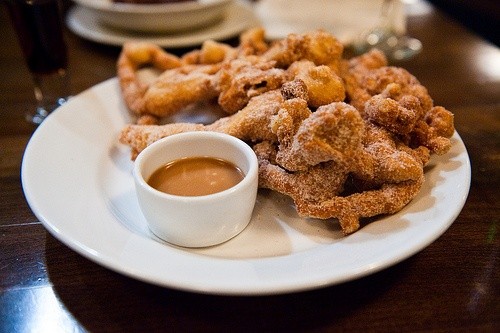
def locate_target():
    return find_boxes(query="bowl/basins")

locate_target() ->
[73,0,235,34]
[134,131,258,247]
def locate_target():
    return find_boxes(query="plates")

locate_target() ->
[67,0,251,47]
[21,68,471,296]
